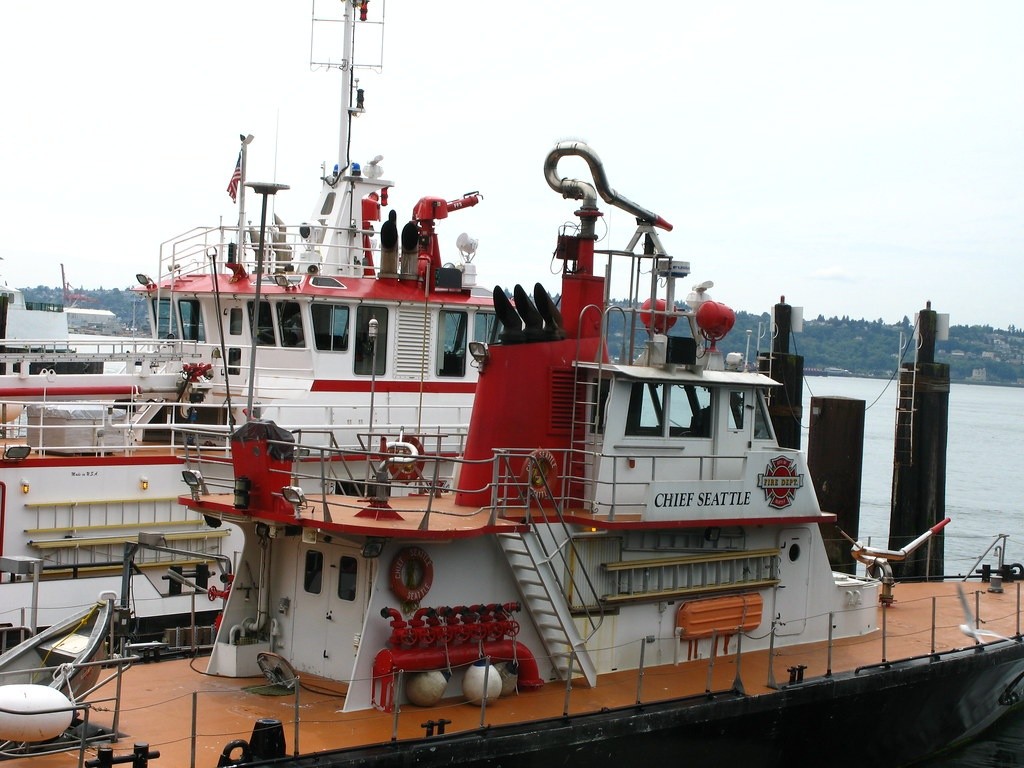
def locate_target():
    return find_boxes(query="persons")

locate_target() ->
[380,210,397,273]
[401,217,418,273]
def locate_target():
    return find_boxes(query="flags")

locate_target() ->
[227,151,242,204]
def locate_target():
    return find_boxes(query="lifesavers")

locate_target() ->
[389,545,434,602]
[388,436,425,483]
[522,448,558,499]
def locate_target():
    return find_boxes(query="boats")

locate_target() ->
[0,0,1024,768]
